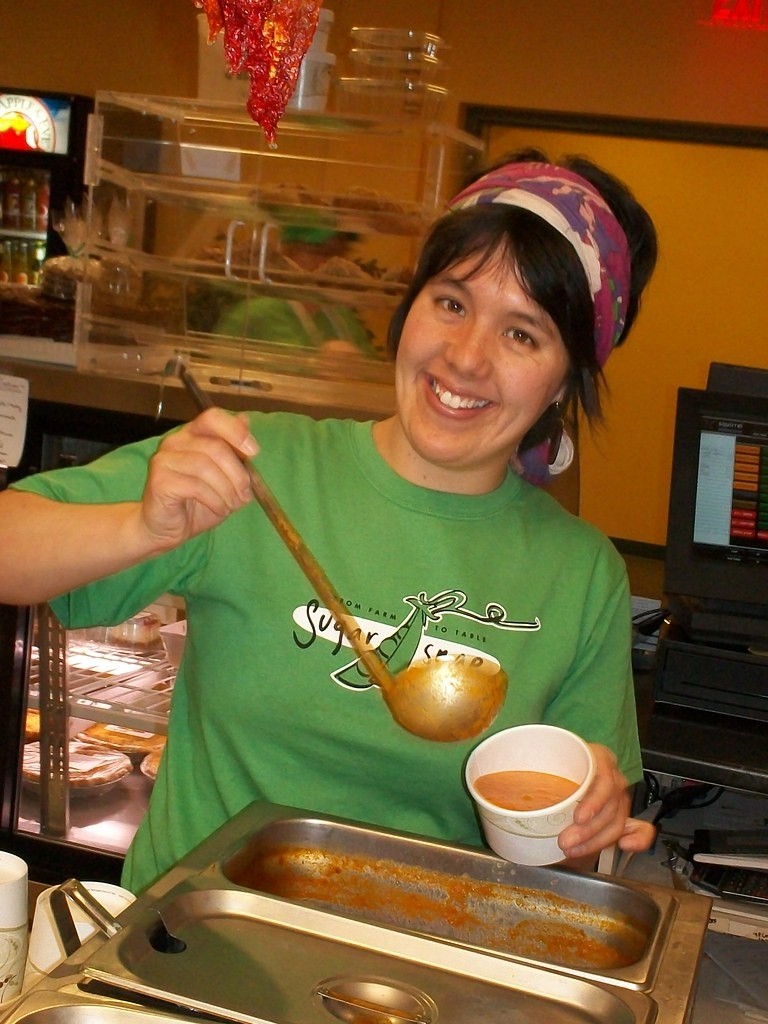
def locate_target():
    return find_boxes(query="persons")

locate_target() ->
[212,207,386,388]
[1,145,658,900]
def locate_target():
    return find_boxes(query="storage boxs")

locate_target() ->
[72,2,486,379]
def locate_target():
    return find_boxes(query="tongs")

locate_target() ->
[49,879,201,1014]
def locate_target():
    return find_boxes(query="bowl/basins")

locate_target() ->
[22,772,129,799]
[71,733,166,786]
[0,849,139,1006]
[196,5,449,127]
[157,619,187,668]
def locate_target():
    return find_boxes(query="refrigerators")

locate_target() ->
[1,85,162,345]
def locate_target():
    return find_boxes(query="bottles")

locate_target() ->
[0,174,50,285]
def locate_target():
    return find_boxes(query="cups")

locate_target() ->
[465,724,594,867]
